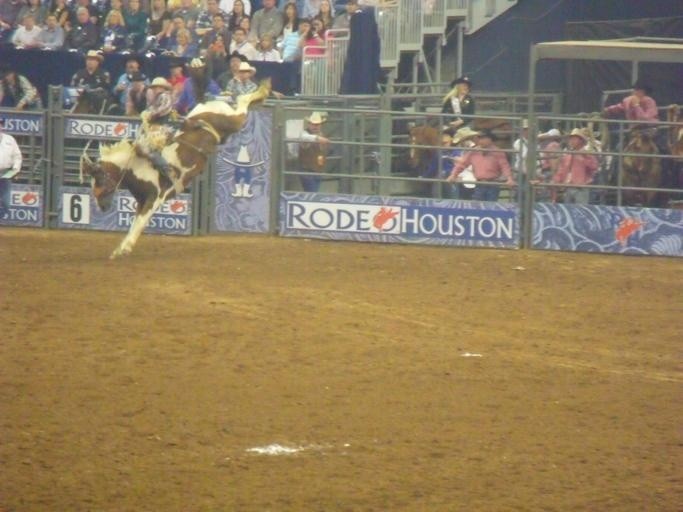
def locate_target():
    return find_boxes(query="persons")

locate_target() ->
[512,81,662,205]
[297,112,331,191]
[419,77,515,199]
[2,0,353,149]
[0,133,24,221]
[340,1,380,94]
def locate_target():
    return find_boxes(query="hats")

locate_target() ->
[305,111,327,124]
[630,77,652,93]
[452,127,480,144]
[82,50,105,62]
[127,72,170,89]
[451,76,471,88]
[237,62,256,76]
[568,128,588,144]
[185,58,206,68]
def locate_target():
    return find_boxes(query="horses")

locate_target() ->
[65,81,121,149]
[616,131,661,206]
[83,78,271,260]
[407,119,514,172]
